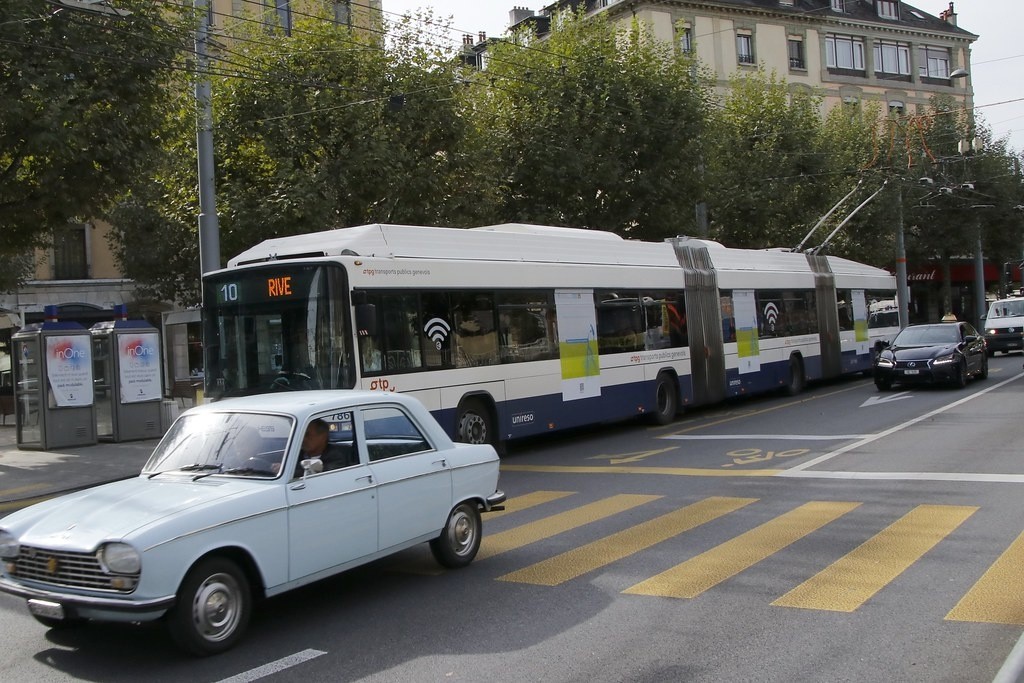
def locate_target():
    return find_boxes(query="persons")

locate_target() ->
[233,425,262,464]
[270,419,331,476]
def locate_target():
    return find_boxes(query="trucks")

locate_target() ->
[980,296,1024,356]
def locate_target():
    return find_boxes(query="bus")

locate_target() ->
[882,257,1022,323]
[197,218,913,482]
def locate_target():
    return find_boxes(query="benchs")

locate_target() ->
[0,395,39,426]
[168,380,204,407]
[331,440,426,461]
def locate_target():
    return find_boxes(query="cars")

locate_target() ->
[1,382,507,659]
[873,313,989,390]
